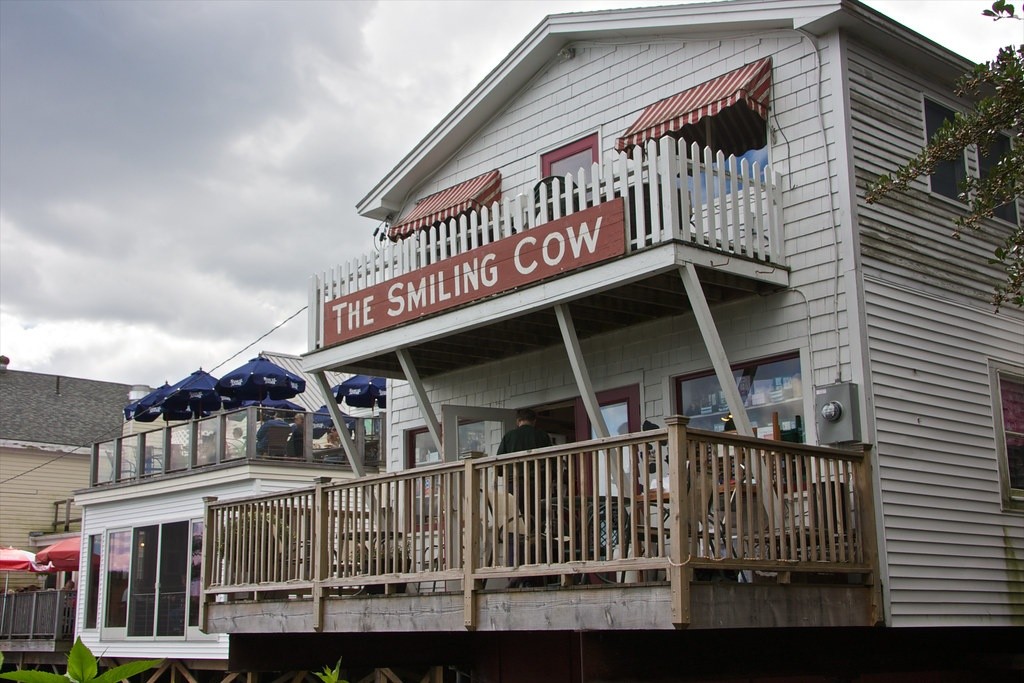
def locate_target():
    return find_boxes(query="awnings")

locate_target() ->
[387,168,502,243]
[614,55,789,160]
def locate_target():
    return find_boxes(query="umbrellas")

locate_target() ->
[0,545,55,636]
[236,395,307,414]
[312,403,365,437]
[215,353,299,429]
[329,373,387,440]
[158,366,229,464]
[35,535,101,583]
[124,381,213,462]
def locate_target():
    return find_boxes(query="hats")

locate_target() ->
[202,431,210,437]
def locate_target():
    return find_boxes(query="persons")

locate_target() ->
[257,409,291,455]
[63,581,77,637]
[495,407,552,588]
[7,587,15,596]
[119,578,151,636]
[226,427,247,459]
[289,412,304,458]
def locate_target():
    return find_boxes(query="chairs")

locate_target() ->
[105,426,292,482]
[618,464,750,586]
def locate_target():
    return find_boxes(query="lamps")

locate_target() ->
[557,48,575,60]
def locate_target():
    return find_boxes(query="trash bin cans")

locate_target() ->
[613,543,644,583]
